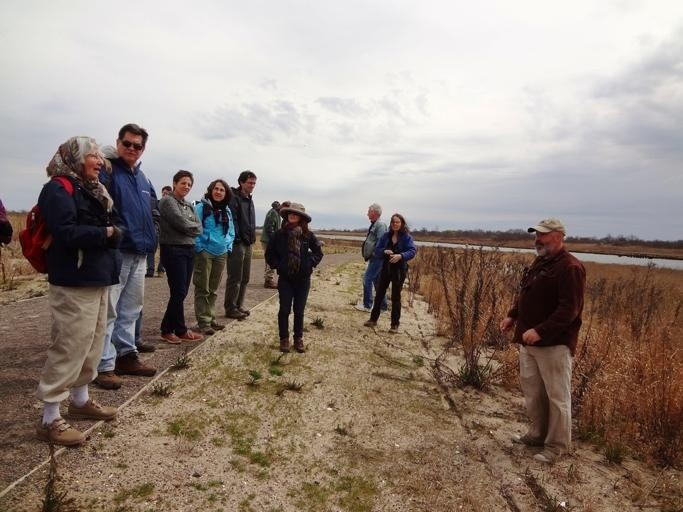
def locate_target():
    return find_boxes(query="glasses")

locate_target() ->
[120,138,145,152]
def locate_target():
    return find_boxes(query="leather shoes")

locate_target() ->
[37,417,86,446]
[69,398,118,420]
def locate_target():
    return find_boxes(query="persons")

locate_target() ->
[260,201,294,290]
[265,203,323,353]
[156,184,173,279]
[499,219,587,464]
[355,203,387,313]
[145,252,155,277]
[228,170,257,318]
[96,123,156,388]
[134,313,155,353]
[364,214,417,335]
[157,169,204,343]
[36,137,117,445]
[194,178,235,335]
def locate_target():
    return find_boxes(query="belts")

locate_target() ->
[159,243,195,249]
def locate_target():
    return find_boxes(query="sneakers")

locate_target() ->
[198,320,225,334]
[264,279,279,289]
[114,354,156,376]
[533,449,571,464]
[93,369,122,389]
[388,326,398,334]
[293,336,306,353]
[279,337,290,353]
[146,269,165,278]
[161,333,181,344]
[354,304,373,313]
[364,320,377,327]
[225,306,251,319]
[136,340,156,353]
[177,330,203,341]
[510,433,546,446]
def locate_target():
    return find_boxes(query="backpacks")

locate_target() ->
[191,200,229,235]
[18,176,74,274]
[381,252,405,283]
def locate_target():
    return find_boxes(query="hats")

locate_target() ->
[279,202,311,223]
[527,218,566,237]
